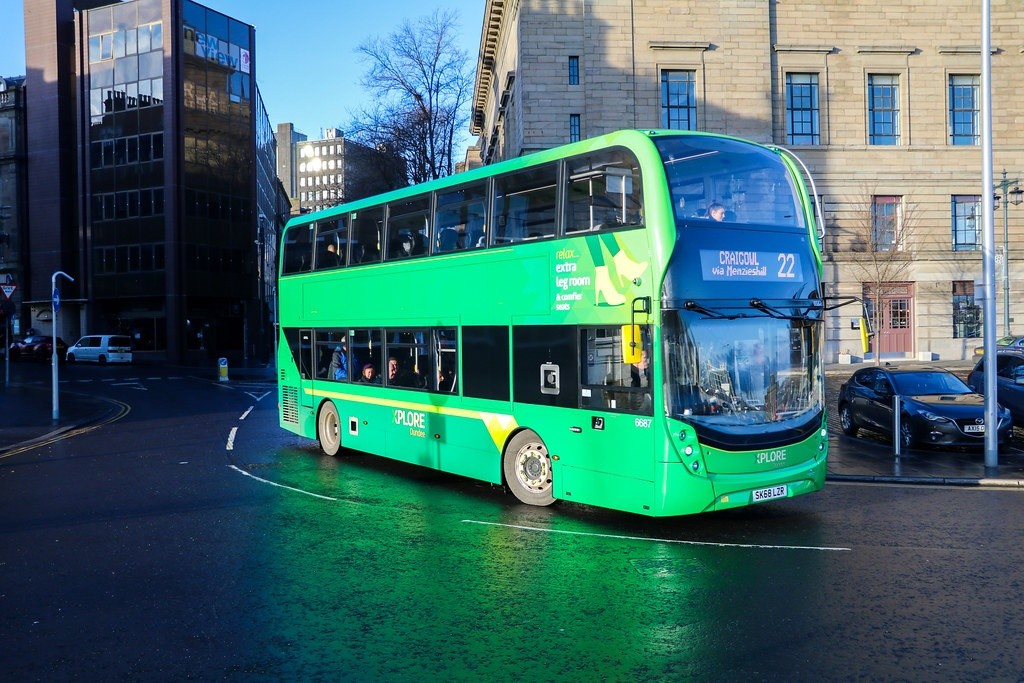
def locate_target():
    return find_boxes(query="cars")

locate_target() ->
[9,335,68,359]
[972,335,1024,367]
[967,344,1024,429]
[838,360,1014,452]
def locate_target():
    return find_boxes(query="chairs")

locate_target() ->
[284,208,744,272]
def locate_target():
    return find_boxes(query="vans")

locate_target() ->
[65,335,134,365]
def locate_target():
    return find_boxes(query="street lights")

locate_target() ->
[991,168,1024,335]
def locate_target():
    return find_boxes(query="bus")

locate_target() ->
[271,126,878,519]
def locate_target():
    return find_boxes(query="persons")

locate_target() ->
[395,228,428,258]
[385,356,428,387]
[708,202,726,222]
[631,351,652,387]
[322,244,342,268]
[317,336,362,381]
[358,364,374,383]
[439,229,485,250]
[439,370,456,391]
